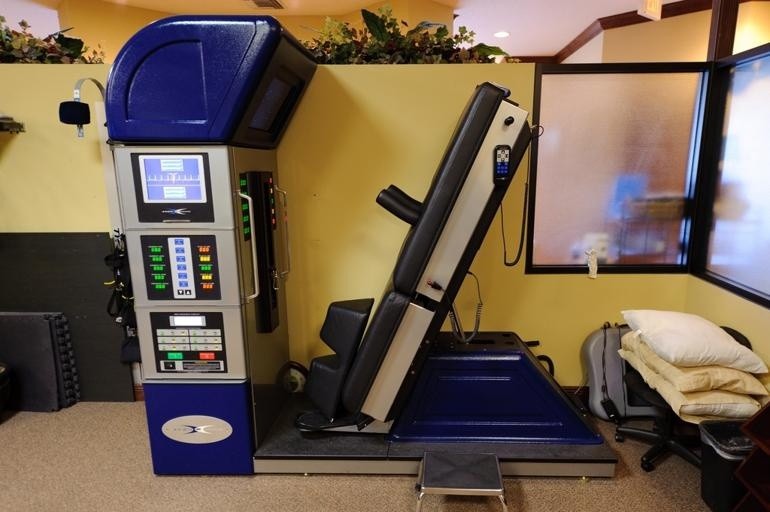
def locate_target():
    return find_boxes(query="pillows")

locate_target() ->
[617,309,769,425]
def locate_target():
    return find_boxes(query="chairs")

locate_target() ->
[615,327,753,473]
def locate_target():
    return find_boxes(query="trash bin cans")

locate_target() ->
[699,419,757,512]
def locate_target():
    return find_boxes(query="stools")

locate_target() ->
[416,452,508,512]
